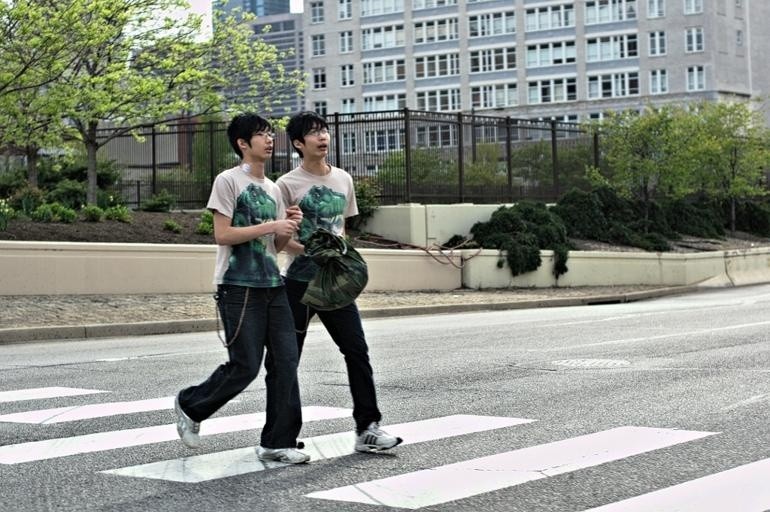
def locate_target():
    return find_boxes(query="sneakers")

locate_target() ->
[174,392,202,450]
[256,445,312,465]
[295,436,306,449]
[352,420,404,452]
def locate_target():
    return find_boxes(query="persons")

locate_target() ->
[274,110,405,453]
[174,111,311,466]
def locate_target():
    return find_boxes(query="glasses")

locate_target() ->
[251,132,275,138]
[304,128,329,137]
